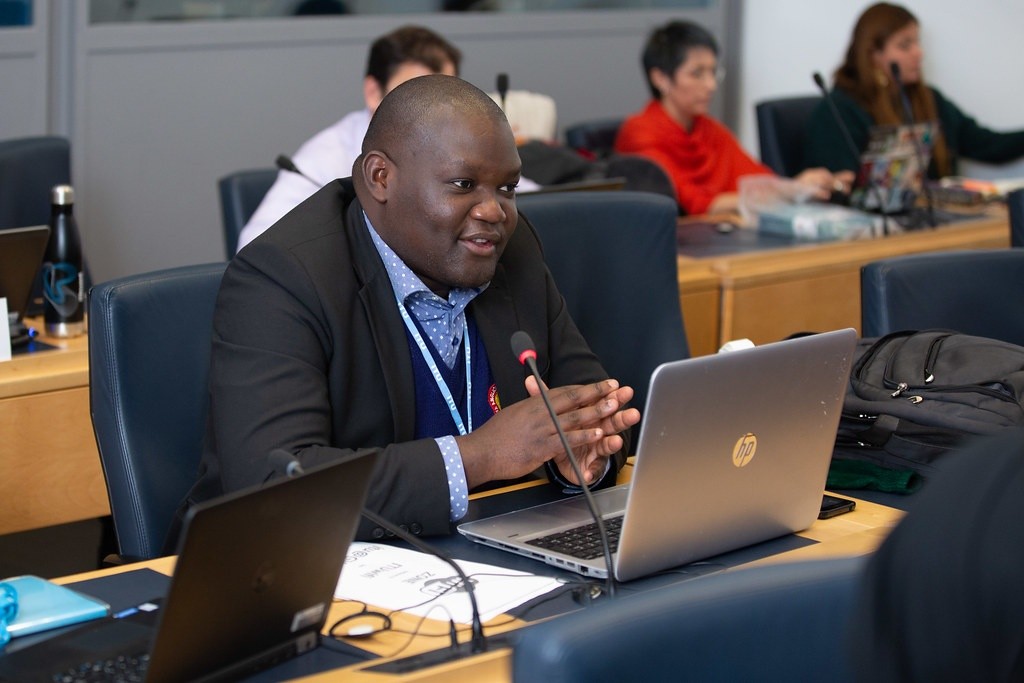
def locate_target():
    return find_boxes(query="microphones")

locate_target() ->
[510,331,617,599]
[267,449,488,653]
[813,73,890,239]
[274,154,324,189]
[497,72,510,111]
[890,64,940,228]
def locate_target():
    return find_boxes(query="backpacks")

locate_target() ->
[777,329,1024,495]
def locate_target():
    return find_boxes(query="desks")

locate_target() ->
[50,457,907,683]
[674,204,1010,359]
[0,312,114,537]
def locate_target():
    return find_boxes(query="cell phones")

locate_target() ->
[818,495,855,518]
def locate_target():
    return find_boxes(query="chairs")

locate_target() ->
[0,134,78,231]
[510,192,691,427]
[755,97,839,177]
[860,251,1024,350]
[563,117,627,158]
[508,558,874,683]
[216,169,278,259]
[88,261,227,560]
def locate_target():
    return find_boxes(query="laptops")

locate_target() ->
[0,446,383,683]
[457,329,859,582]
[0,226,50,324]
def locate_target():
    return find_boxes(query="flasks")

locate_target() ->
[44,185,86,337]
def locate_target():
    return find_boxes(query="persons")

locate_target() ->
[863,421,1024,683]
[237,27,542,255]
[814,3,1024,211]
[614,19,855,215]
[163,76,641,556]
